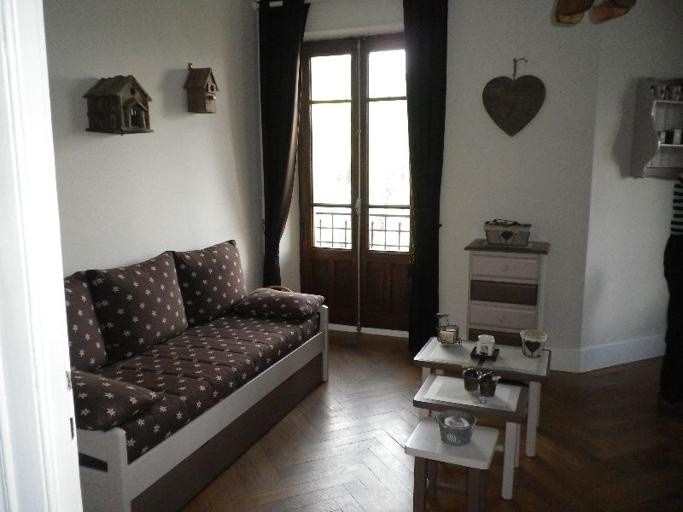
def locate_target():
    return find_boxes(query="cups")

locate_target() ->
[477,375,497,398]
[463,372,482,392]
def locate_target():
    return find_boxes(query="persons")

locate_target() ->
[656,166,682,412]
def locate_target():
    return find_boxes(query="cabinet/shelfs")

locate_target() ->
[629,77,683,181]
[464,238,551,347]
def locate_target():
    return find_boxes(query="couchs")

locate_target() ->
[64,237,331,512]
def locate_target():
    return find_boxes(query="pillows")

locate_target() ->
[70,368,162,430]
[232,285,326,324]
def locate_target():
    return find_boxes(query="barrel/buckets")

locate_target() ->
[435,411,477,446]
[520,328,548,357]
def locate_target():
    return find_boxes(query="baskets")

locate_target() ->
[484,223,531,246]
